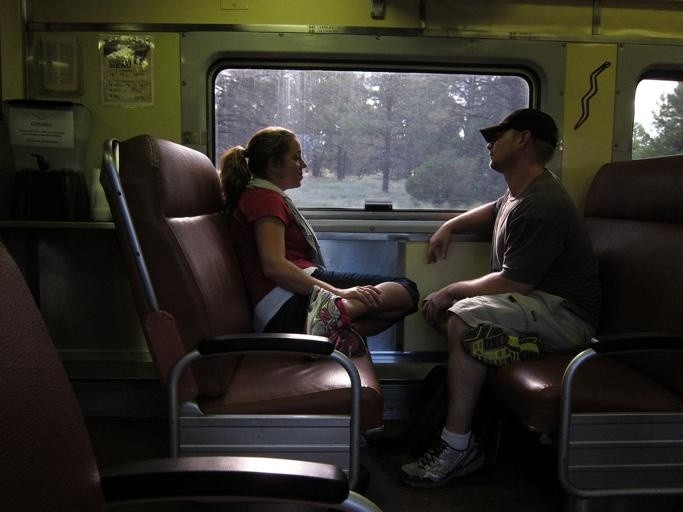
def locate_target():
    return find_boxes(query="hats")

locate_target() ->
[479,108,558,148]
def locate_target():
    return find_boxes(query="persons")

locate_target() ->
[219,125,423,361]
[393,105,614,494]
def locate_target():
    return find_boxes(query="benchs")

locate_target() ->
[0,239,382,512]
[490,155,683,512]
[98,135,382,477]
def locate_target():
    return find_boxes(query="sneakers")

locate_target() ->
[401,431,482,487]
[306,284,361,360]
[461,321,541,365]
